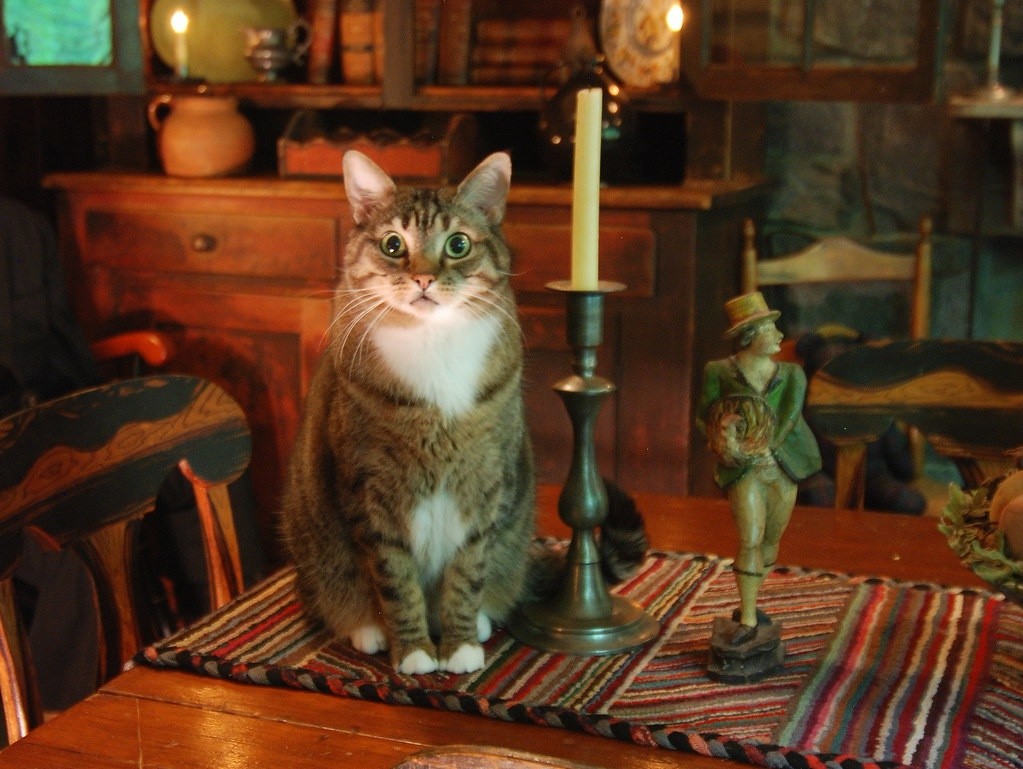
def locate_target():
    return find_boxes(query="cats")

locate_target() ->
[279,149,537,676]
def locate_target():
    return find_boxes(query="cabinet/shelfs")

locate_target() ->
[44,169,771,490]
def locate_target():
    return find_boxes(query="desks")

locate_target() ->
[1,490,1023,769]
[691,333,1023,519]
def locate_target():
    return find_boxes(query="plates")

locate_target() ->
[599,0,680,92]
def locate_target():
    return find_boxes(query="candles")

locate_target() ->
[571,89,600,287]
[173,8,193,80]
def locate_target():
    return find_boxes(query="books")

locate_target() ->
[307,1,593,84]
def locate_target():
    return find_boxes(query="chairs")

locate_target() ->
[1,367,253,748]
[741,219,934,508]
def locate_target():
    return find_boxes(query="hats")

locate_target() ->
[598,0,684,89]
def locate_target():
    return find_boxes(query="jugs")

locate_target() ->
[148,86,255,178]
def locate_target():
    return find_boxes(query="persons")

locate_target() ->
[695,292,825,648]
